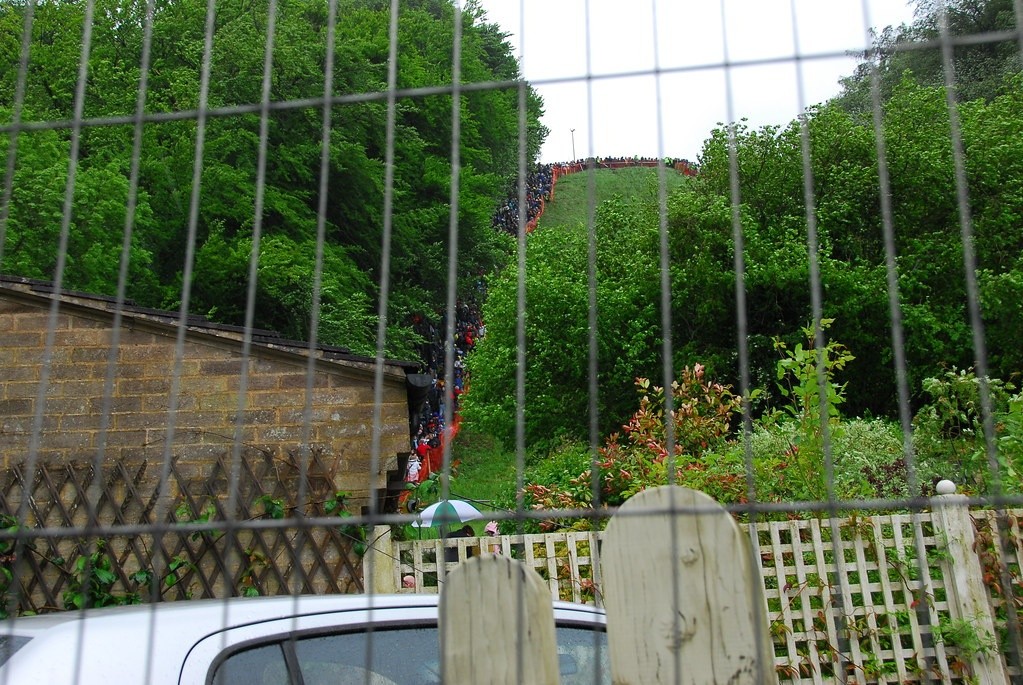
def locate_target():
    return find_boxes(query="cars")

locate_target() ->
[1,593,608,685]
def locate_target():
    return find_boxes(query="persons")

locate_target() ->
[395,153,697,483]
[483,520,502,556]
[436,524,476,575]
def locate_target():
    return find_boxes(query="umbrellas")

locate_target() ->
[411,499,482,536]
